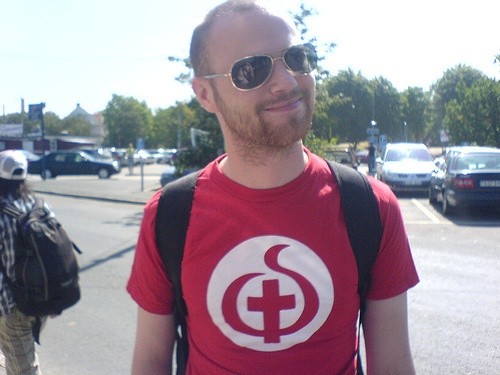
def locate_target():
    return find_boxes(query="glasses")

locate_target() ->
[203,42,319,92]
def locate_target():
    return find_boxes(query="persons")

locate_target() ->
[126,0,421,375]
[366,143,376,168]
[0,149,54,375]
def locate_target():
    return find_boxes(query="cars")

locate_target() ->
[27,148,176,179]
[375,142,437,193]
[325,146,362,168]
[430,146,500,217]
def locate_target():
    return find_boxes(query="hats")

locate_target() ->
[0,149,28,181]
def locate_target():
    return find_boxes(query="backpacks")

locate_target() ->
[0,196,83,347]
